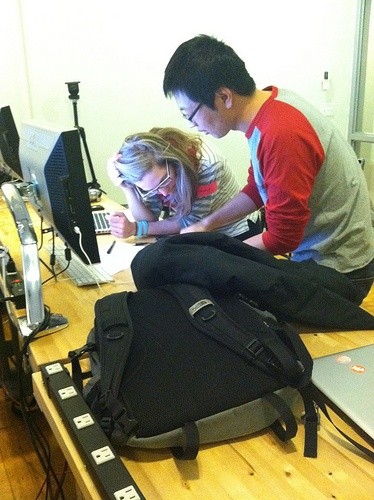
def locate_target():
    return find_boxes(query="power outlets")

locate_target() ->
[39,361,146,500]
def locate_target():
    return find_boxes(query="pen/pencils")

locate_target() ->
[107,239,117,254]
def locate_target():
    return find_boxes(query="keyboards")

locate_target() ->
[45,242,116,287]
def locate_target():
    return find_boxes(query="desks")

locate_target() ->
[0,192,374,500]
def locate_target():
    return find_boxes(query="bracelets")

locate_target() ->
[135,221,148,237]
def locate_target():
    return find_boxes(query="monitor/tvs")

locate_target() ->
[0,106,102,341]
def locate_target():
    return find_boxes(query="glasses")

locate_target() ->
[135,159,172,202]
[182,101,204,128]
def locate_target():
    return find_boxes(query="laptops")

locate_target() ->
[309,345,374,451]
[91,209,137,235]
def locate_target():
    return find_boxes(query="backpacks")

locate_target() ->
[68,282,374,460]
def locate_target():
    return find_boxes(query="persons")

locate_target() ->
[163,34,374,309]
[109,128,262,242]
